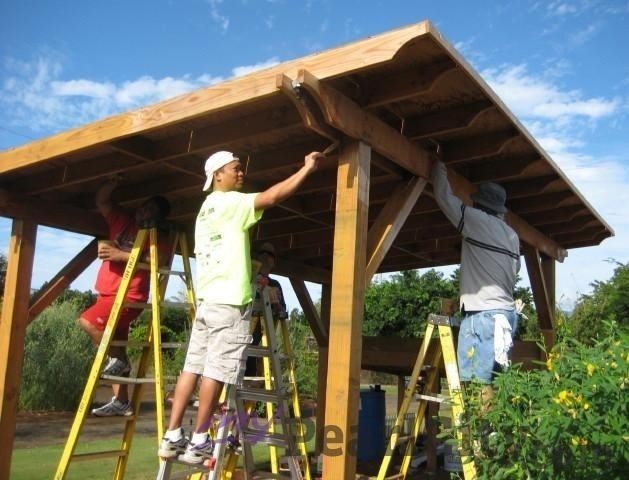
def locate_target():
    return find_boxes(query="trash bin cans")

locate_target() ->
[356,385,386,477]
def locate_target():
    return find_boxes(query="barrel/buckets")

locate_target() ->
[357,385,386,460]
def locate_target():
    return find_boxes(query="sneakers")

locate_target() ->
[91,395,135,417]
[183,434,215,464]
[157,427,190,458]
[226,433,243,453]
[100,357,133,376]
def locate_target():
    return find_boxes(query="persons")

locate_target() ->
[430,150,521,459]
[157,146,327,464]
[246,239,288,374]
[80,175,171,417]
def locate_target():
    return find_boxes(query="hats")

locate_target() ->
[202,149,240,194]
[251,243,275,257]
[468,181,509,214]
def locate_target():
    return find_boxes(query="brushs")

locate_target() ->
[107,175,127,180]
[322,139,341,155]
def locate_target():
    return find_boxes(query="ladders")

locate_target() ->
[54,215,311,480]
[376,312,478,480]
